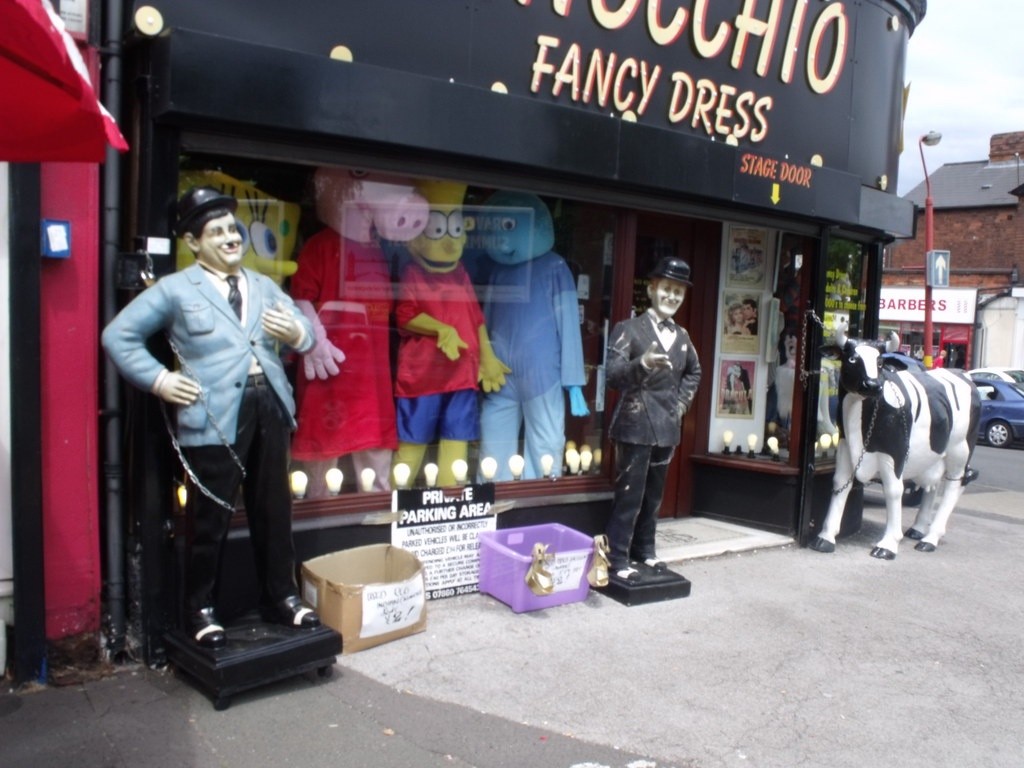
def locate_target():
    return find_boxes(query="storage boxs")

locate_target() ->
[476,522,597,614]
[299,543,427,656]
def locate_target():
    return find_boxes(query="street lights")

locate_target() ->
[919,130,941,369]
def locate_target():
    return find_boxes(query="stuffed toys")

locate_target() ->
[477,192,589,487]
[176,171,299,287]
[391,180,512,489]
[292,166,429,499]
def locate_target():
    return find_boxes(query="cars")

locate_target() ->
[965,370,1024,382]
[971,379,1024,448]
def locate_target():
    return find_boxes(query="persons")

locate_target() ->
[101,190,319,648]
[767,325,834,438]
[726,299,759,336]
[606,256,701,584]
[933,350,947,369]
